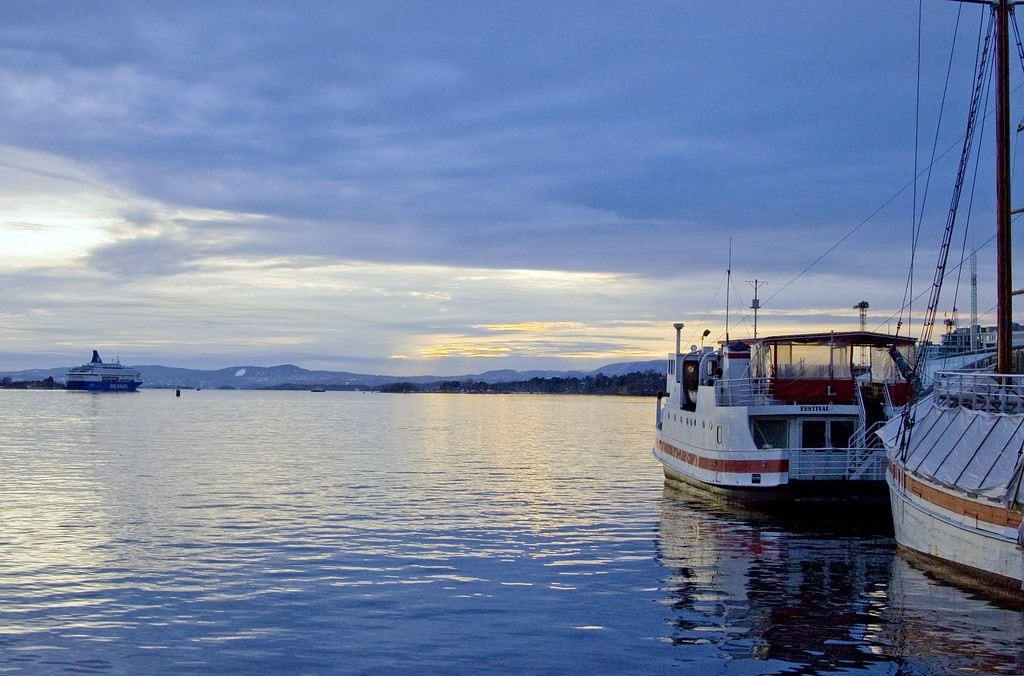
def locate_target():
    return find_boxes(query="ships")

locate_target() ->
[66,355,143,392]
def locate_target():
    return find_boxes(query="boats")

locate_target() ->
[874,0,1024,595]
[652,236,922,502]
